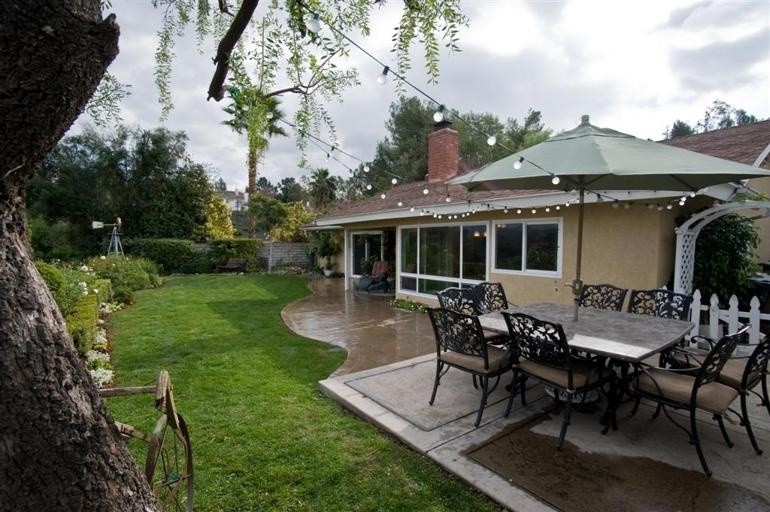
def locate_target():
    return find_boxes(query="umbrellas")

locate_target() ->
[437,111,770,321]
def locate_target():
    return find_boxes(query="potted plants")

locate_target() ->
[313,234,343,278]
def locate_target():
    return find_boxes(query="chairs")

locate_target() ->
[353,258,392,294]
